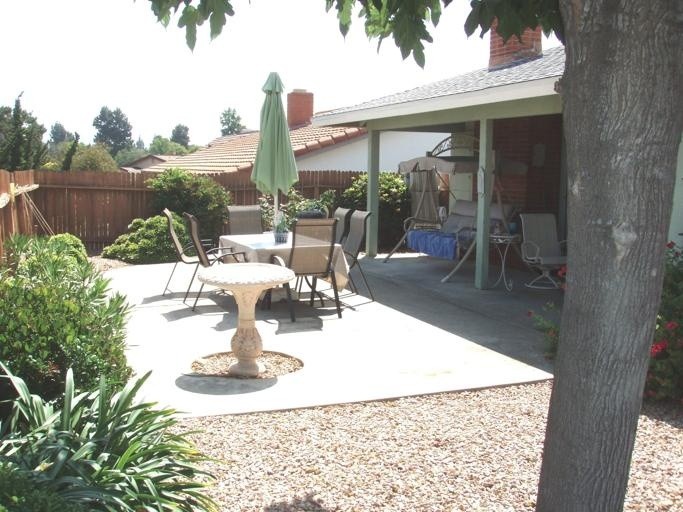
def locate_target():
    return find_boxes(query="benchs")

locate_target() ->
[401,200,515,262]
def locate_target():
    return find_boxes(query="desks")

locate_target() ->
[487,235,521,292]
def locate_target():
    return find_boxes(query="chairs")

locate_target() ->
[162,203,373,324]
[518,212,569,289]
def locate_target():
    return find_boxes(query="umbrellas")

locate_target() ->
[251,72,299,225]
